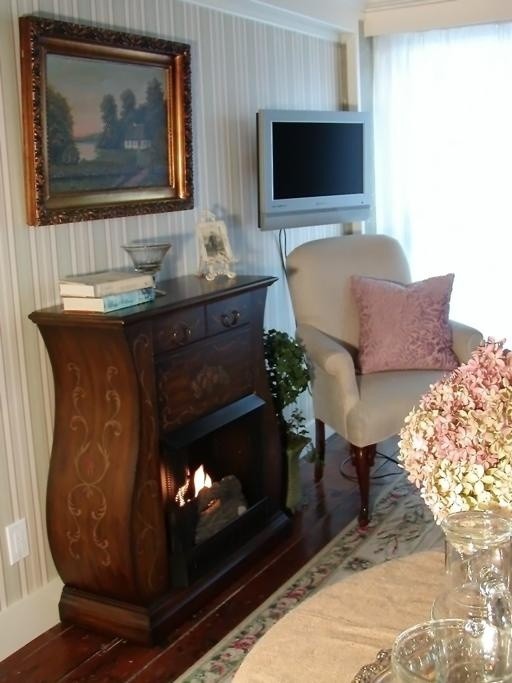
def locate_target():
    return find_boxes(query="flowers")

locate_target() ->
[399,340,512,550]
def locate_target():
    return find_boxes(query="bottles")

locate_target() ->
[432,511,511,628]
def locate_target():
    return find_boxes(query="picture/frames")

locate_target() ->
[18,13,194,225]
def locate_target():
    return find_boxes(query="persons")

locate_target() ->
[206,230,222,252]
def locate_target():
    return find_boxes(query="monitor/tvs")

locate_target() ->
[256,108,374,232]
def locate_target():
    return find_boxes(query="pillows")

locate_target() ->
[351,272,459,374]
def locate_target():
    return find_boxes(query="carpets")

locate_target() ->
[173,475,447,682]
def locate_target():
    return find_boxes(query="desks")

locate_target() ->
[230,550,446,683]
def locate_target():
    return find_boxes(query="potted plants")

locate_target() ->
[262,329,327,516]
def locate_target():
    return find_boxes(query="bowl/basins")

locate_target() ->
[391,618,511,682]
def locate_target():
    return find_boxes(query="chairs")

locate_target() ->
[286,235,483,527]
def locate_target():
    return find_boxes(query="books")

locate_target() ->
[60,269,154,296]
[60,289,154,313]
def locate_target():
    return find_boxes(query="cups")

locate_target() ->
[120,241,172,298]
